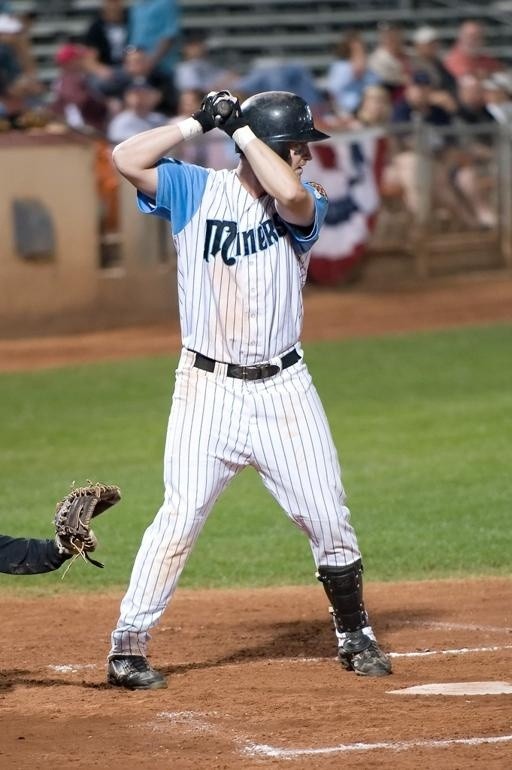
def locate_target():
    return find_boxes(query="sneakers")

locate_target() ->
[108,653,169,691]
[336,629,392,676]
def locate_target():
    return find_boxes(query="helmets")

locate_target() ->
[234,91,331,153]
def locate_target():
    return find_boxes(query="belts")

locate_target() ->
[186,349,302,381]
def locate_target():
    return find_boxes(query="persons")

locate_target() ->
[107,90,392,690]
[0,529,98,575]
[1,0,181,146]
[174,20,512,229]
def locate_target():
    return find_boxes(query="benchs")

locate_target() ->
[0,0,512,279]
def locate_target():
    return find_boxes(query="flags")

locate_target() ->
[299,129,388,286]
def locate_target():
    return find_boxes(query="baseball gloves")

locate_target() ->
[56,482,120,581]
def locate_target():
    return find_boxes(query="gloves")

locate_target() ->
[192,89,250,138]
